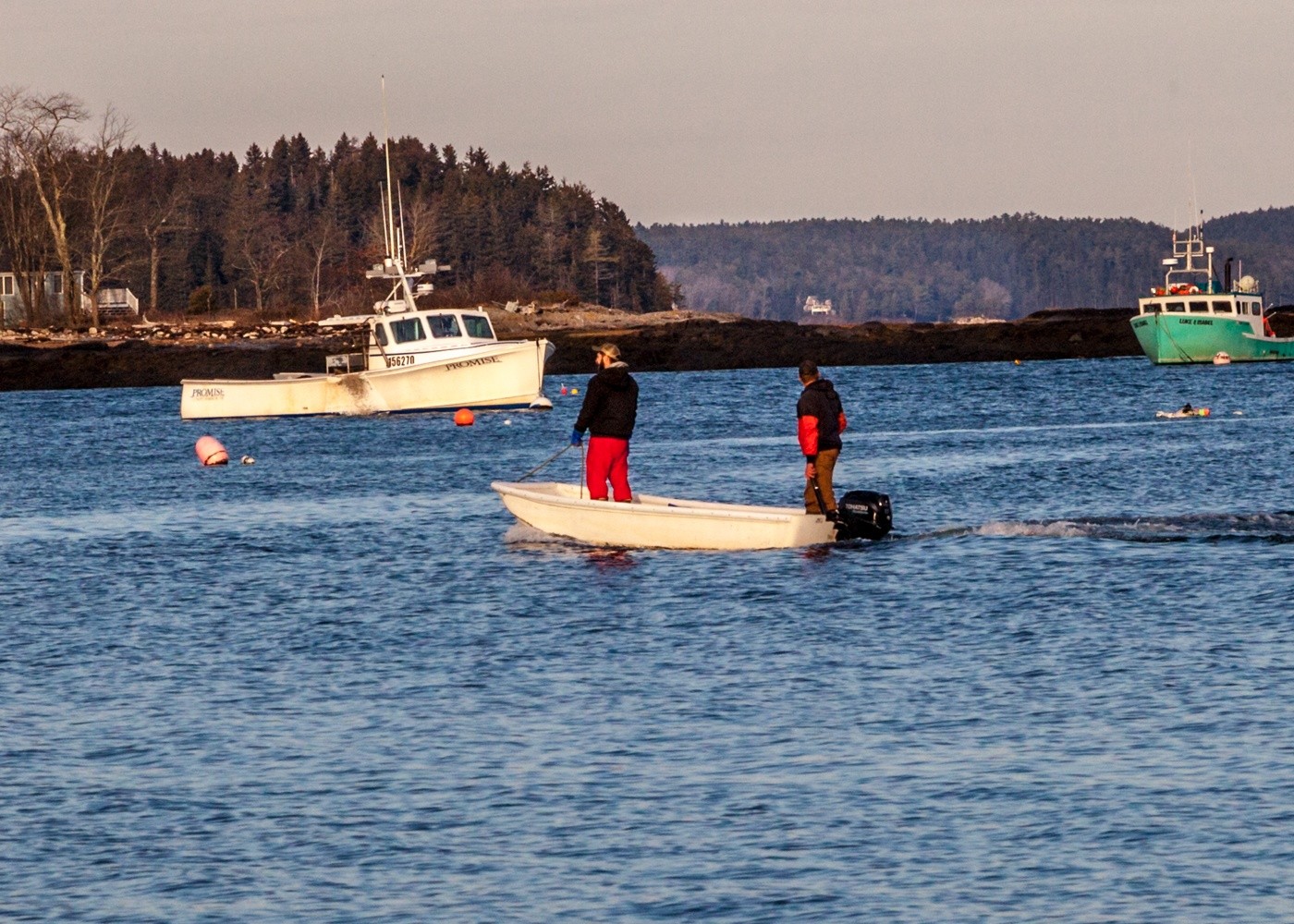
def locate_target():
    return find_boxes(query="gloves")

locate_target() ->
[570,431,583,447]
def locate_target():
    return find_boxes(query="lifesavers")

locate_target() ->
[1188,286,1199,295]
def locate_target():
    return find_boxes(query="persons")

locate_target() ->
[571,341,640,502]
[796,360,847,515]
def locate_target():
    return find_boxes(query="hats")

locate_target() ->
[591,342,621,361]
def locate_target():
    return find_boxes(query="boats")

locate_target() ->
[1155,403,1211,418]
[490,473,894,548]
[1128,183,1294,365]
[178,73,557,419]
